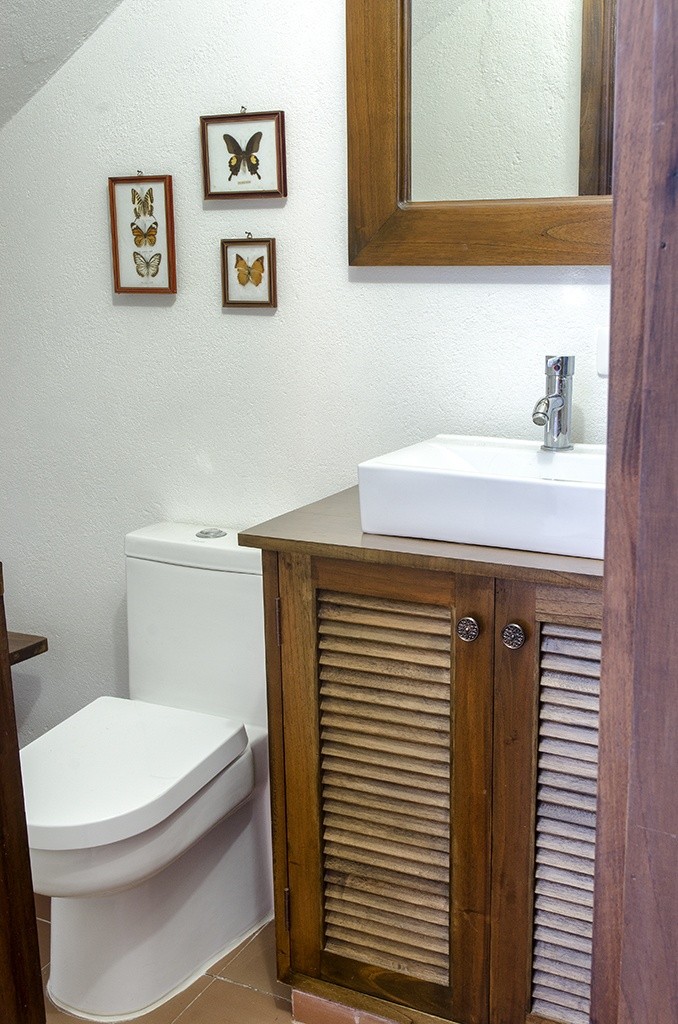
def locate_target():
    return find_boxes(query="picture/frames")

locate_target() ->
[108,174,177,293]
[200,111,288,200]
[221,237,277,307]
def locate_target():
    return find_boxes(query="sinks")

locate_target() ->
[356,432,607,561]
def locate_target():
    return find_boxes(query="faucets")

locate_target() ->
[530,352,577,454]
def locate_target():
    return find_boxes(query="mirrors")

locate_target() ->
[346,0,617,267]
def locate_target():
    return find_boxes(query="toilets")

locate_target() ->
[17,520,276,1019]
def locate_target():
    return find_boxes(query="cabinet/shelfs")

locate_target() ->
[259,550,607,1024]
